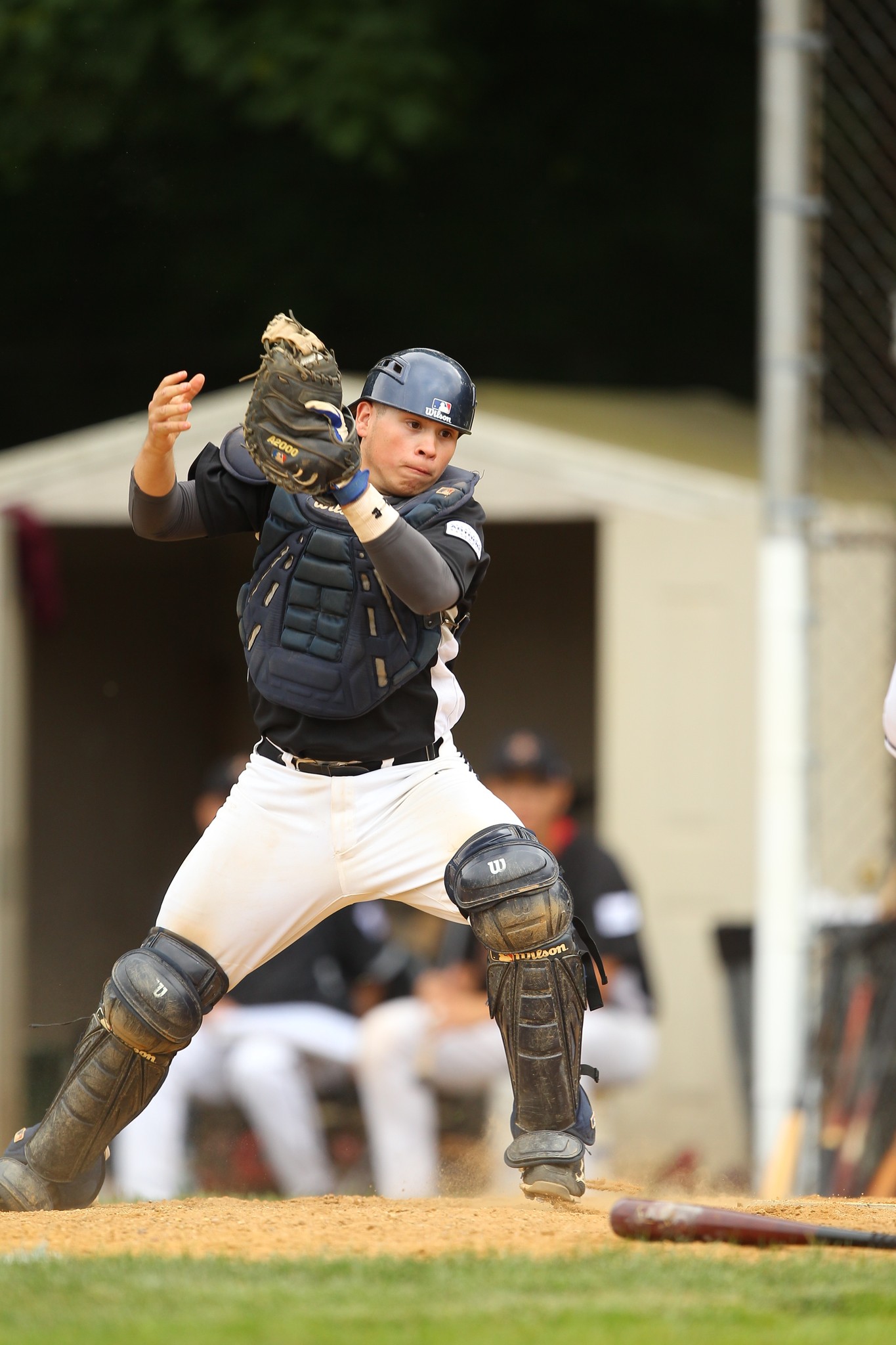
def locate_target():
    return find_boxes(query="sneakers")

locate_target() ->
[521,1162,584,1200]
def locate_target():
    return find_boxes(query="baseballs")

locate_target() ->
[297,349,324,367]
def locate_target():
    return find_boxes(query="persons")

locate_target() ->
[815,662,896,1195]
[109,756,414,1201]
[350,731,657,1198]
[2,310,610,1210]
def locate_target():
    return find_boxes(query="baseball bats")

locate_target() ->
[609,1199,896,1251]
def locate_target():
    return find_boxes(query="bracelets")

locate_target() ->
[343,482,399,543]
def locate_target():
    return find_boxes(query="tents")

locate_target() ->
[1,369,896,1194]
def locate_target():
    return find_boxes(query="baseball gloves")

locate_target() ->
[243,312,363,496]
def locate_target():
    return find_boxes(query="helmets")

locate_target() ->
[361,348,476,435]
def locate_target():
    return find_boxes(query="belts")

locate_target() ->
[256,735,443,777]
